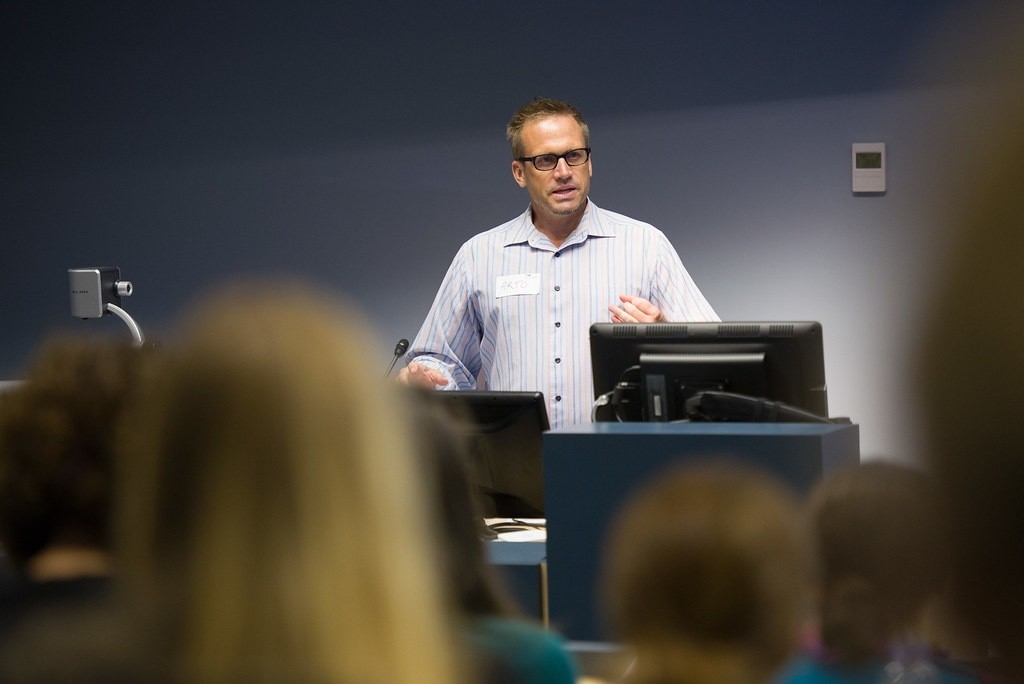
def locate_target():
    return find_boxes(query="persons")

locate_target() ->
[2,276,1020,684]
[386,99,724,429]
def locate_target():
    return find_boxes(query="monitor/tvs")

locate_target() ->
[589,320,829,424]
[437,390,555,519]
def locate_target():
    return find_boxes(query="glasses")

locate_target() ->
[517,148,591,172]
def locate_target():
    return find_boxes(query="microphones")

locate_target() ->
[385,339,409,384]
